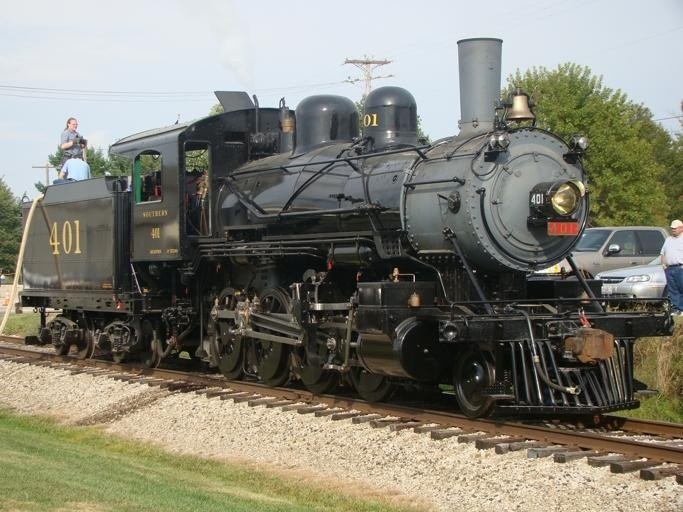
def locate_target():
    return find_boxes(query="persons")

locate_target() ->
[52,148,92,185]
[58,117,89,180]
[659,219,682,316]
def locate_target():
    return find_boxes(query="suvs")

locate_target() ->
[533,225,669,298]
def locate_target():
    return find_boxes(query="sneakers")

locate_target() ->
[671,310,683,317]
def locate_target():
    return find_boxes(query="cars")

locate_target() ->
[593,255,673,312]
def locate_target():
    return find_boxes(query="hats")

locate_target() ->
[670,220,683,228]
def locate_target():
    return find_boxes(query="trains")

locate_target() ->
[15,35,675,429]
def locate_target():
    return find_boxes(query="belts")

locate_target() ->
[669,264,683,267]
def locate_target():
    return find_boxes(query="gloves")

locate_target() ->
[73,134,87,144]
